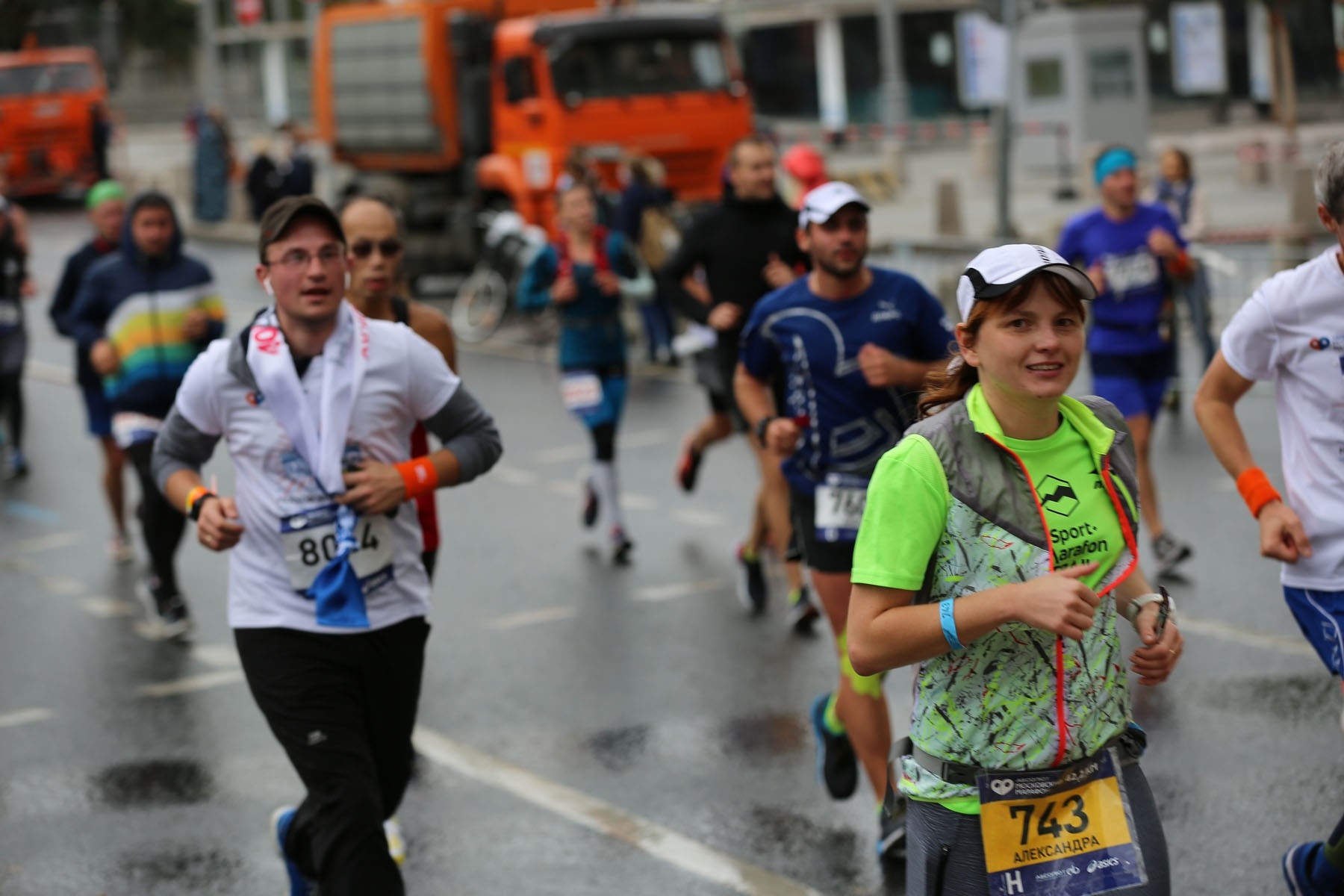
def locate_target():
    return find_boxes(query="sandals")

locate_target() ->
[584,482,599,528]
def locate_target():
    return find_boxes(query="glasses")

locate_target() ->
[278,245,340,267]
[352,240,398,259]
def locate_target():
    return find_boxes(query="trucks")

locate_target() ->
[0,46,123,201]
[309,0,765,284]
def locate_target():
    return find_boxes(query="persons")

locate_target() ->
[0,188,34,491]
[1056,146,1216,580]
[469,142,698,371]
[77,95,347,235]
[667,134,829,642]
[733,179,971,859]
[1195,135,1344,896]
[46,179,146,566]
[69,190,227,641]
[327,184,460,868]
[515,168,656,566]
[149,195,503,896]
[843,242,1184,896]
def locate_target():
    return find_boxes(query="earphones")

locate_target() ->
[344,271,353,292]
[262,274,273,297]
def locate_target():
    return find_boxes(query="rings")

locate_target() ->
[1169,648,1179,661]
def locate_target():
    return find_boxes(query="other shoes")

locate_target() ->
[272,806,323,896]
[1153,540,1192,578]
[612,534,633,567]
[115,531,134,563]
[788,583,821,627]
[1282,839,1326,896]
[735,541,767,608]
[134,576,192,645]
[681,445,704,492]
[813,689,860,802]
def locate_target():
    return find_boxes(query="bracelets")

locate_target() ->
[397,453,437,499]
[939,597,968,653]
[1237,468,1283,517]
[183,487,215,522]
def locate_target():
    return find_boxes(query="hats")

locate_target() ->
[798,181,871,230]
[259,196,345,263]
[956,245,1099,326]
[87,178,124,208]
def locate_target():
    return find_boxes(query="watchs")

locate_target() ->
[1124,593,1177,623]
[756,416,775,445]
